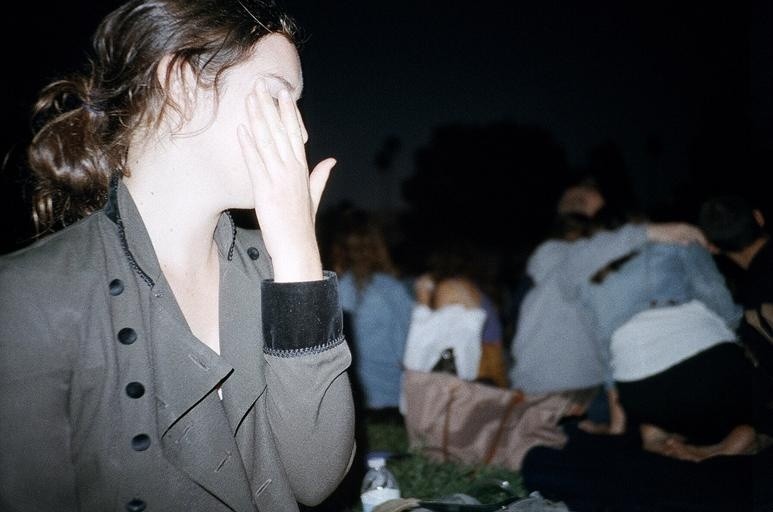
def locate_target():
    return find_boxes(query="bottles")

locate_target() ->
[361,457,401,511]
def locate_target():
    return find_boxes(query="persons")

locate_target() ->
[0,0,356,512]
[331,186,772,462]
